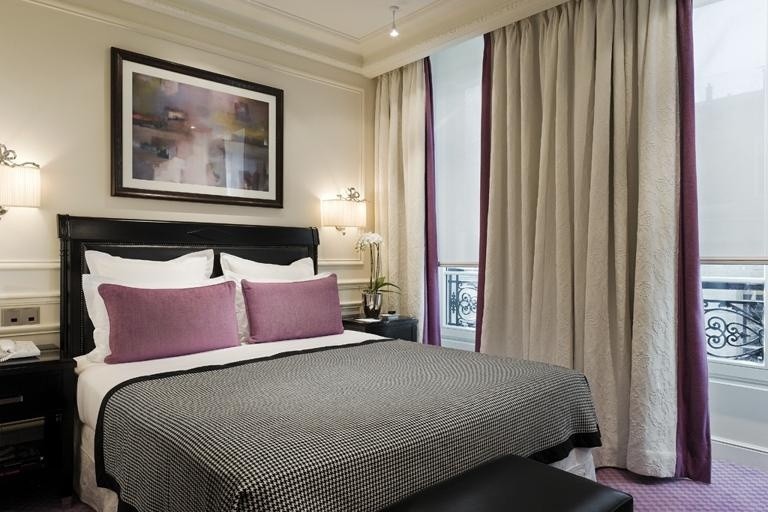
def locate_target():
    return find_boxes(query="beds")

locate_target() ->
[57,213,606,512]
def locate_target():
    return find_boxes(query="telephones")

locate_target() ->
[0,339,41,362]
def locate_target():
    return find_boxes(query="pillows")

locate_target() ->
[224,271,331,341]
[217,250,318,283]
[96,282,243,365]
[79,275,242,371]
[241,274,352,346]
[84,246,216,287]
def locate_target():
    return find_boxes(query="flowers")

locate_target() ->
[356,233,392,293]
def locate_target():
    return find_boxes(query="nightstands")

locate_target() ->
[342,314,418,341]
[0,344,59,488]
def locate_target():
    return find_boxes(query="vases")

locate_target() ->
[362,289,382,317]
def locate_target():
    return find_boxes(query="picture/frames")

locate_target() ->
[110,47,284,208]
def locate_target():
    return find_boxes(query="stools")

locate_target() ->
[374,453,634,512]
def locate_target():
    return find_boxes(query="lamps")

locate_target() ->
[389,6,402,39]
[0,144,42,219]
[316,187,368,236]
